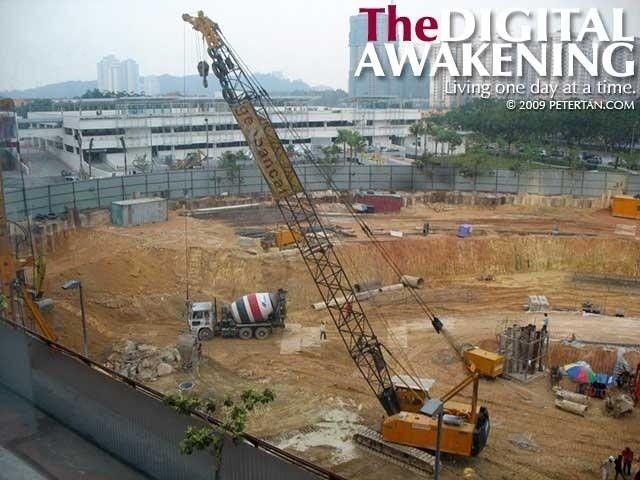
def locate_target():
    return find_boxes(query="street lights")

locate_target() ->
[88,136,95,177]
[61,276,91,361]
[204,117,211,158]
[119,135,129,176]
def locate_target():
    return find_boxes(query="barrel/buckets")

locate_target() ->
[457,224,472,237]
[552,226,560,236]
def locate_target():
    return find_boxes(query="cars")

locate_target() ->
[364,145,376,153]
[484,139,638,170]
[64,175,81,183]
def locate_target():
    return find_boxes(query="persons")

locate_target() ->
[622,447,633,476]
[600,456,615,480]
[344,302,352,322]
[614,455,626,480]
[320,322,326,341]
[541,313,549,334]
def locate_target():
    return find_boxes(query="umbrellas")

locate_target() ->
[564,360,597,384]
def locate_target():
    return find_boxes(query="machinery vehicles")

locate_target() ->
[188,286,292,344]
[174,6,506,477]
[13,258,55,312]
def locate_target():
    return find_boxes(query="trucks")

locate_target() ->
[258,224,302,249]
